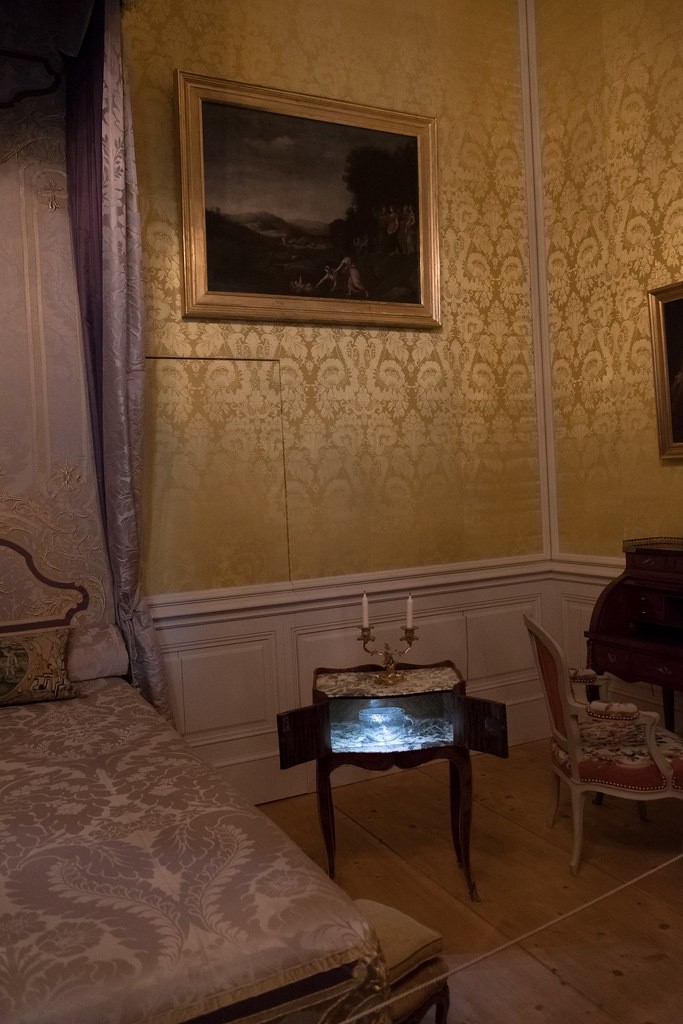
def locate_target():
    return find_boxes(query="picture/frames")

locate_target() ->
[646,280,683,459]
[172,69,442,329]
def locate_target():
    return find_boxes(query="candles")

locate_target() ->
[406,592,413,628]
[361,590,369,627]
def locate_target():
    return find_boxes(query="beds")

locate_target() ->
[0,470,384,1024]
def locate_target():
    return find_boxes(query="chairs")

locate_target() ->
[522,611,683,878]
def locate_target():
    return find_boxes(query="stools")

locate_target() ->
[355,897,450,1024]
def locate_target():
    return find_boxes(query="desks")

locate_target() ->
[278,662,508,900]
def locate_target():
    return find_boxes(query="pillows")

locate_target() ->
[0,627,88,706]
[66,623,130,682]
